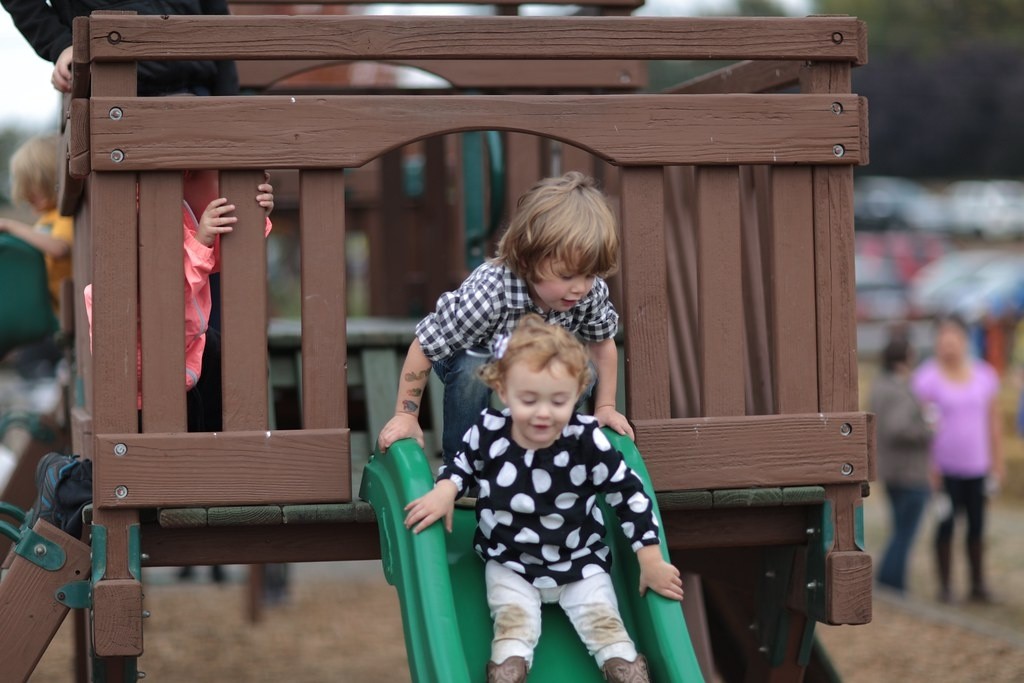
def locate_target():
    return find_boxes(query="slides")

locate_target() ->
[358,422,708,683]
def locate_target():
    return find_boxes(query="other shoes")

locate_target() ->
[938,585,988,604]
[874,571,907,594]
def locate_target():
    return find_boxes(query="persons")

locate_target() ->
[379,177,635,506]
[0,137,74,489]
[866,340,934,594]
[911,320,1003,603]
[404,313,684,683]
[0,0,241,102]
[32,166,272,533]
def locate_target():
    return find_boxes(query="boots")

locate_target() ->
[486,657,529,683]
[602,655,648,683]
